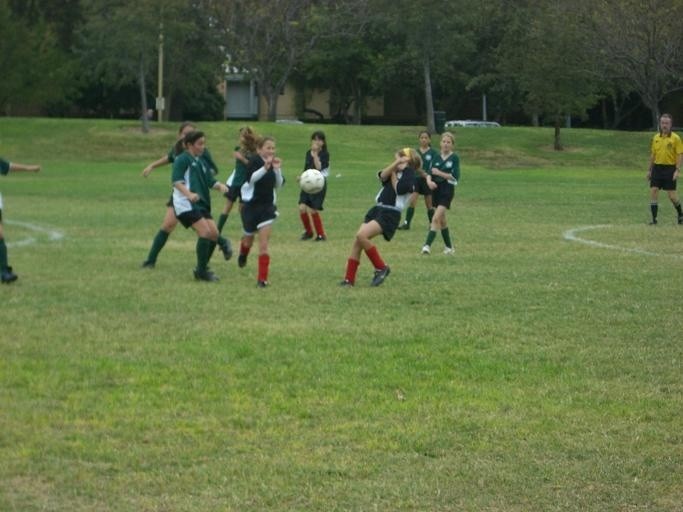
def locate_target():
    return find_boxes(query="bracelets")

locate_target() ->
[675,167,681,171]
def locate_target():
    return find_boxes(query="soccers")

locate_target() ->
[299,169,325,194]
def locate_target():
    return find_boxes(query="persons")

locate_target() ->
[397,131,439,230]
[646,113,683,224]
[216,126,263,241]
[0,157,41,284]
[171,131,220,282]
[297,130,329,242]
[421,131,460,255]
[140,121,232,268]
[336,147,420,287]
[238,136,286,290]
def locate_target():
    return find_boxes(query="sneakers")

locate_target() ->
[421,244,431,254]
[678,213,683,224]
[192,266,220,282]
[441,244,455,255]
[370,264,390,287]
[142,261,155,268]
[314,235,326,242]
[397,224,410,230]
[219,240,233,260]
[0,266,18,284]
[648,222,657,225]
[336,279,354,287]
[298,231,313,241]
[256,280,269,288]
[238,254,247,269]
